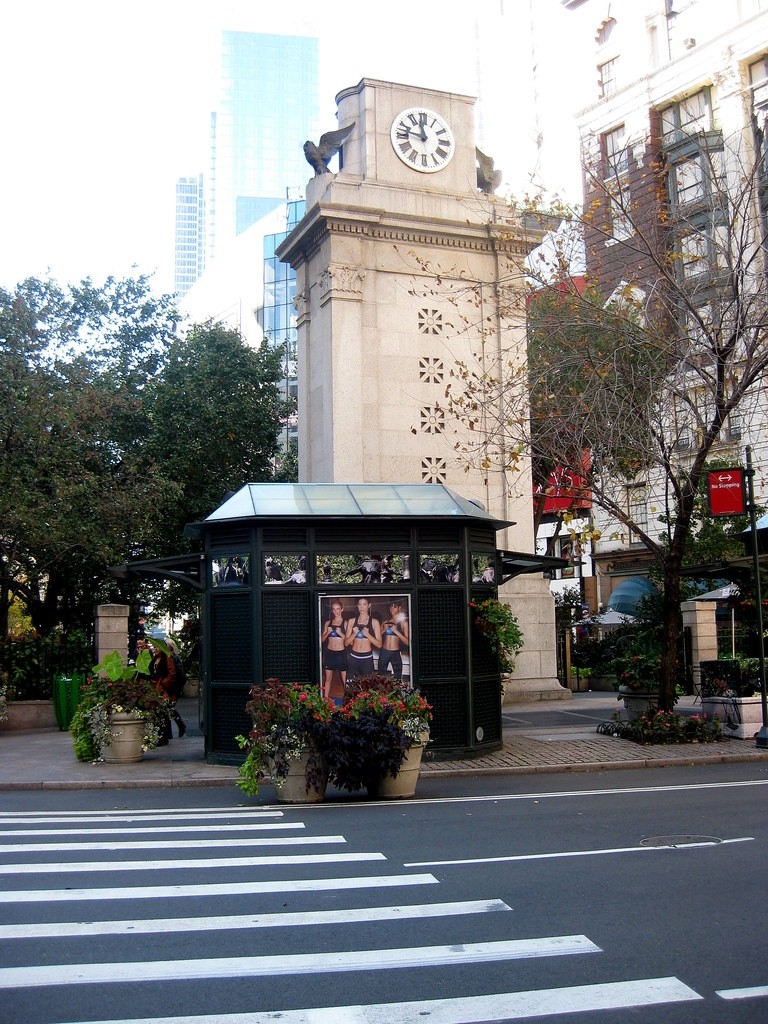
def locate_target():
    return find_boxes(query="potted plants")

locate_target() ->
[570,666,617,691]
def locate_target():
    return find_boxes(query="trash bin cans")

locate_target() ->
[53,672,94,730]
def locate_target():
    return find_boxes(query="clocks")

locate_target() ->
[390,107,455,174]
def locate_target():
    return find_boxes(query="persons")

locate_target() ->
[322,599,409,700]
[561,540,574,577]
[137,637,186,746]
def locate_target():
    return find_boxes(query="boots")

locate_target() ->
[155,719,169,747]
[170,710,186,738]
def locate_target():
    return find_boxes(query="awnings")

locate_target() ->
[608,575,657,616]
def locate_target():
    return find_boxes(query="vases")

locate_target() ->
[701,697,763,741]
[500,673,511,706]
[266,742,329,803]
[102,713,147,763]
[365,729,429,799]
[619,685,660,722]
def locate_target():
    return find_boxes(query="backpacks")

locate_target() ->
[160,653,188,702]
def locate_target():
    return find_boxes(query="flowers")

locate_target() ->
[233,677,434,795]
[620,655,661,688]
[705,678,735,698]
[68,675,176,761]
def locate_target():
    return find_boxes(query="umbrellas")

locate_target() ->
[687,582,741,660]
[571,608,652,631]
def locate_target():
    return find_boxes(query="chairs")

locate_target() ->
[689,666,709,707]
